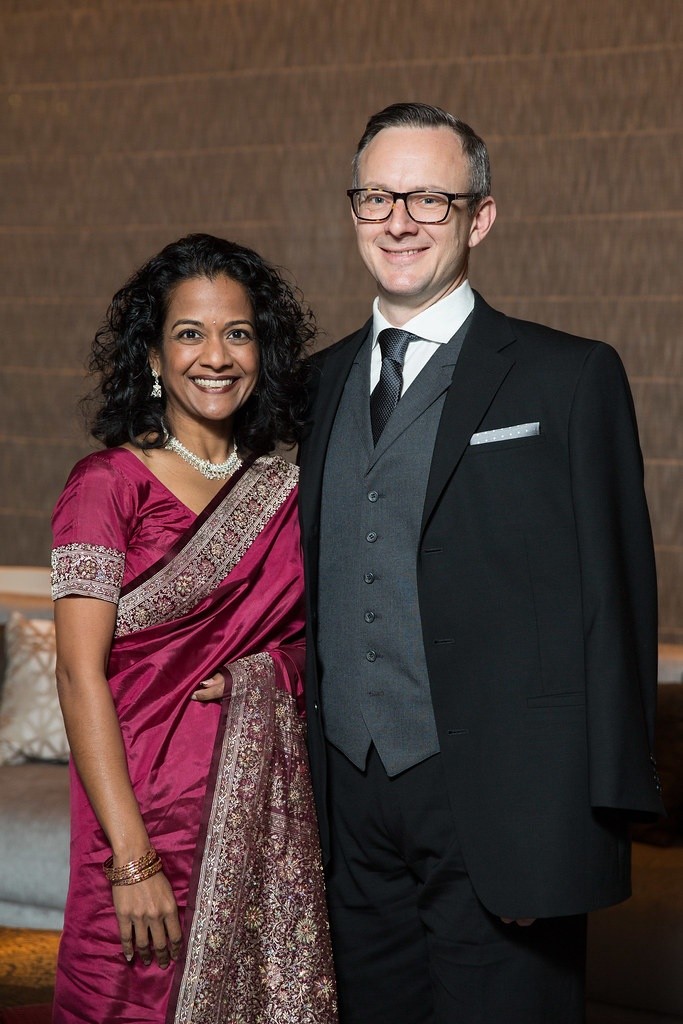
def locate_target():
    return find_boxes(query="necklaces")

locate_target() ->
[159,415,244,480]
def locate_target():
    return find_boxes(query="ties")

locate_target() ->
[370,328,422,448]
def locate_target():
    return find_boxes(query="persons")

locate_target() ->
[279,102,658,1024]
[48,229,337,1024]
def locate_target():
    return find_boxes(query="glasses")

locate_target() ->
[347,187,480,223]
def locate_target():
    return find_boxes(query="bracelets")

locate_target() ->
[103,846,162,887]
